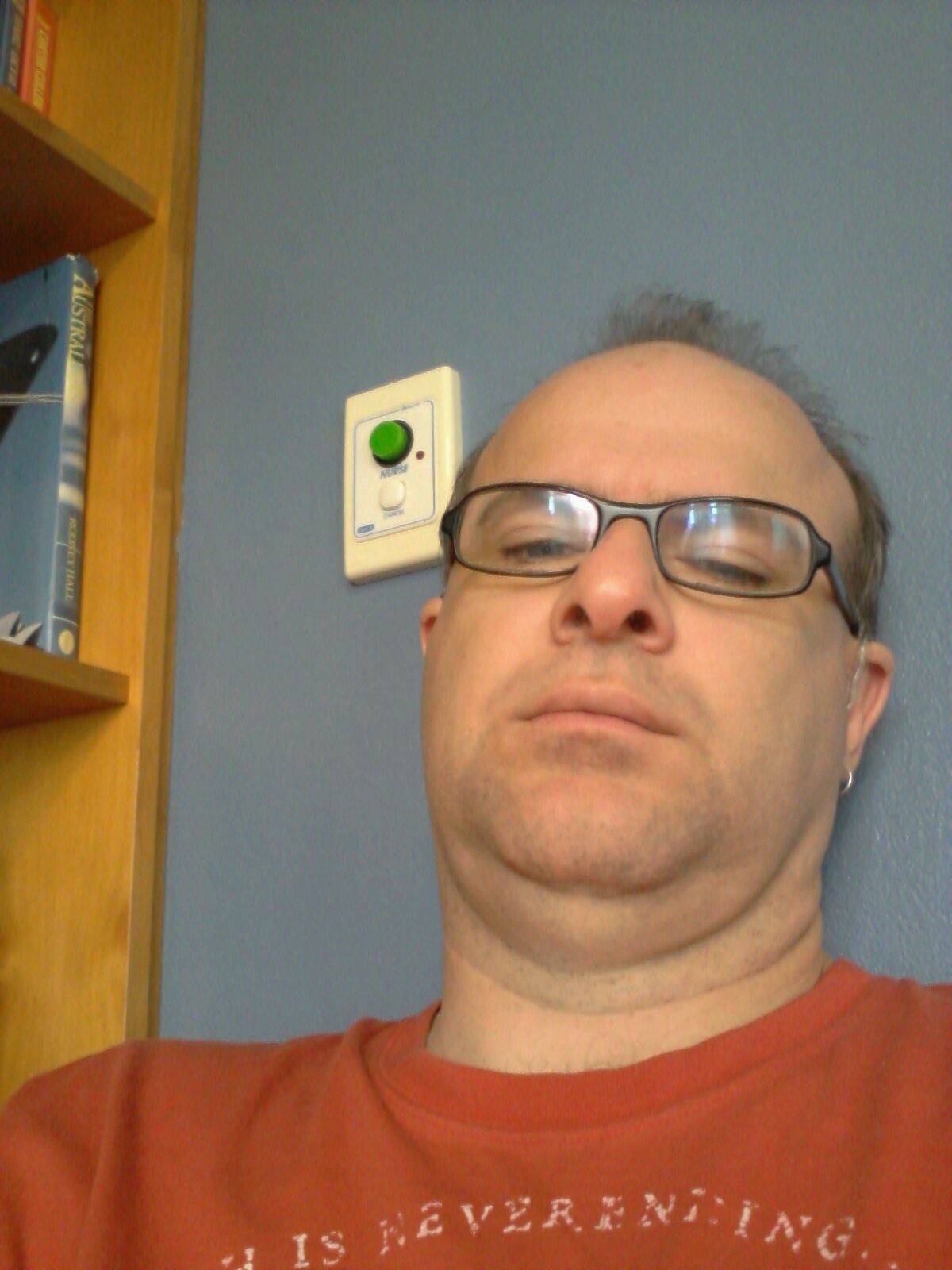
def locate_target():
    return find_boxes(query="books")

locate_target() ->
[0,0,59,119]
[0,254,100,661]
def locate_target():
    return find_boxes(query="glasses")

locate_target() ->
[438,482,859,638]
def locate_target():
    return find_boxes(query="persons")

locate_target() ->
[0,285,952,1270]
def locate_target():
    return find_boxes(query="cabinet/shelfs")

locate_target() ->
[0,0,207,1075]
